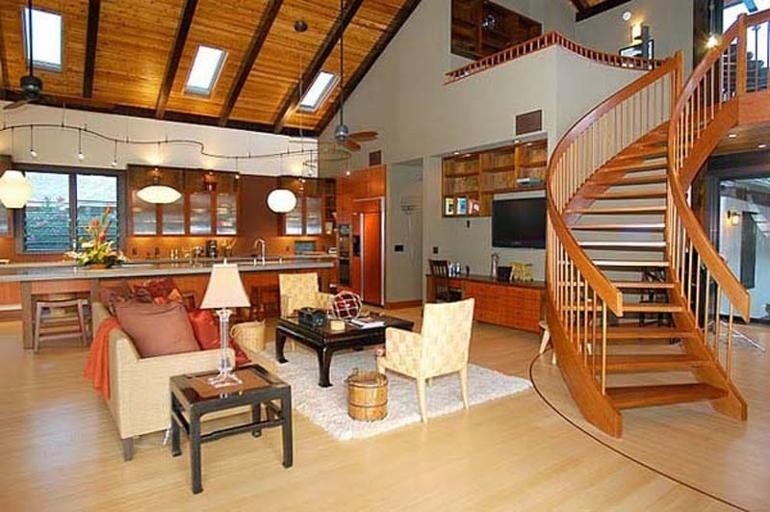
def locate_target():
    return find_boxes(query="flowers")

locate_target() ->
[62,207,131,266]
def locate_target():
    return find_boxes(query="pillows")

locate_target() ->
[110,303,199,353]
[186,309,249,363]
[150,290,183,306]
[134,276,182,302]
[99,279,134,315]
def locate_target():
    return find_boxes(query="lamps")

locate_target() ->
[200,257,251,388]
[136,167,183,204]
[727,210,740,225]
[1,170,32,209]
[266,182,296,214]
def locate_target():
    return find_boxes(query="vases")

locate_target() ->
[90,262,114,269]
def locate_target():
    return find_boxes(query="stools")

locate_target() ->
[34,298,88,353]
[538,321,593,365]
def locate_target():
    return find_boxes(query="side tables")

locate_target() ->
[169,364,293,493]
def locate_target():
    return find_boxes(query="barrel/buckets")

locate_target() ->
[346,369,388,422]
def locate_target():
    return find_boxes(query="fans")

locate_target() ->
[2,75,43,108]
[287,125,378,152]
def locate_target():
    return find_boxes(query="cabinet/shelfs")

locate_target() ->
[316,165,384,306]
[0,253,335,349]
[0,261,118,311]
[440,140,547,218]
[426,274,546,344]
[450,0,542,64]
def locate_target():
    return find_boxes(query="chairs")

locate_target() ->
[429,258,461,301]
[377,298,482,421]
[249,284,279,320]
[279,273,336,351]
[184,292,197,308]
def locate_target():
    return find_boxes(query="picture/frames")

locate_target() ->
[514,110,541,135]
[445,198,454,215]
[468,199,479,214]
[458,197,466,214]
[369,148,382,166]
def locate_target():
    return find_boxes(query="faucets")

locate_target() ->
[254,237,266,266]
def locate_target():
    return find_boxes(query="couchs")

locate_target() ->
[92,291,277,462]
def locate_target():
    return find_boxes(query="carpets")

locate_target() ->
[259,341,532,442]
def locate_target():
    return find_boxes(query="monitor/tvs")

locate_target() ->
[491,197,545,249]
